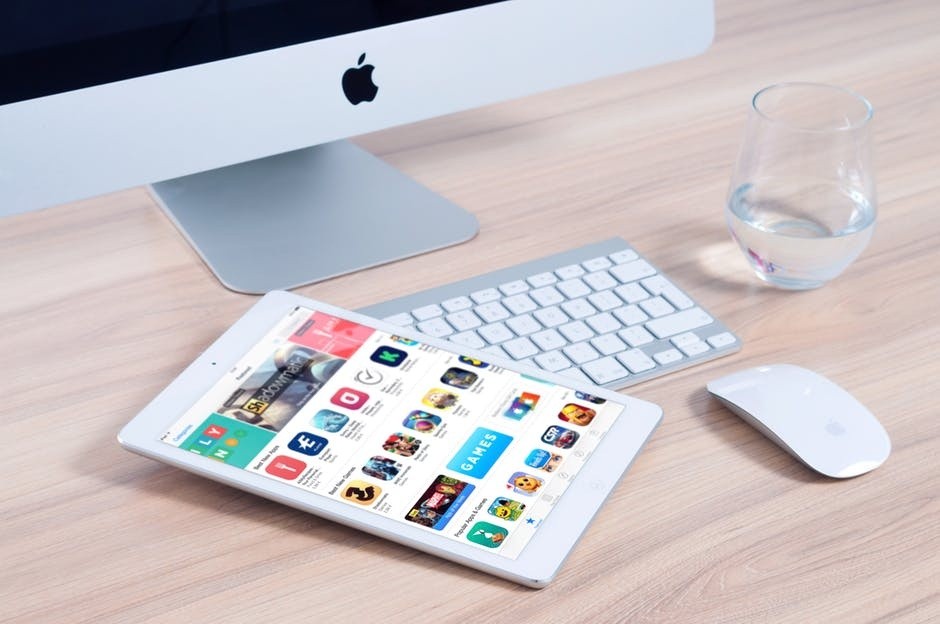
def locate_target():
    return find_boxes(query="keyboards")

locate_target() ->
[349,236,746,392]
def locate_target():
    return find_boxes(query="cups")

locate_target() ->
[725,82,882,289]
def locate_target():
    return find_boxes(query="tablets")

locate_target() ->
[118,290,666,588]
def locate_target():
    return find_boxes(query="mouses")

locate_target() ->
[706,362,890,478]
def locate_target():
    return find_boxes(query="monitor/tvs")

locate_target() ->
[0,0,715,295]
